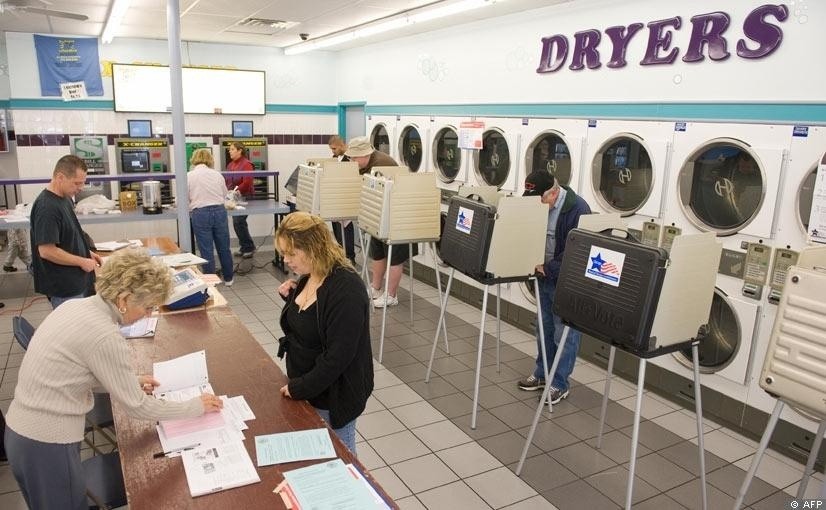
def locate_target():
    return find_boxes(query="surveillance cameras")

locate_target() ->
[299,33,310,42]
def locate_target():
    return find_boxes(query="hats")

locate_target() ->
[522,169,554,196]
[343,137,375,158]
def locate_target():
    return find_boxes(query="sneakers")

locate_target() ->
[518,374,545,390]
[224,276,234,286]
[366,285,382,299]
[235,248,256,258]
[3,267,17,272]
[538,387,569,405]
[370,291,398,308]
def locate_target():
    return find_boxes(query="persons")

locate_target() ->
[329,135,356,267]
[187,149,234,287]
[273,212,374,458]
[4,252,223,509]
[30,156,103,309]
[517,169,591,406]
[226,141,256,259]
[343,138,418,309]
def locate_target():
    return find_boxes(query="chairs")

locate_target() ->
[14,317,116,447]
[76,436,127,510]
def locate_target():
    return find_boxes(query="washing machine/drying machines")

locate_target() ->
[365,114,826,475]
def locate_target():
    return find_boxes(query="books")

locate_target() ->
[151,350,222,438]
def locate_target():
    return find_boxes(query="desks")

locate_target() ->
[0,200,291,311]
[91,237,399,510]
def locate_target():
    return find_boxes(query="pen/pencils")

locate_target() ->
[198,385,204,394]
[152,442,201,459]
